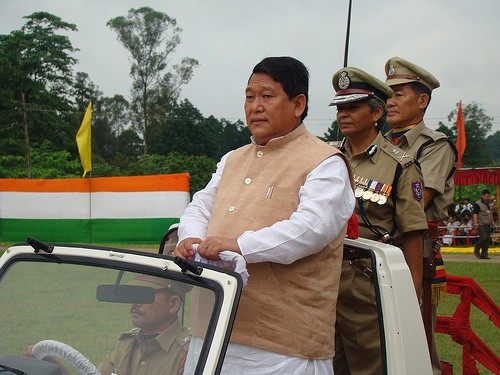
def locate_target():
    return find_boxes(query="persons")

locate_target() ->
[383,57,459,375]
[22,274,192,375]
[437,189,500,259]
[172,56,355,375]
[329,66,428,375]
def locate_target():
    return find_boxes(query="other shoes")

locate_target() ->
[480,252,489,259]
[474,250,480,258]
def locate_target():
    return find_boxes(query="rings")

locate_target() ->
[172,252,174,255]
[203,246,207,249]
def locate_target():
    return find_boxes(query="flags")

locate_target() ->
[456,101,466,168]
[76,100,92,178]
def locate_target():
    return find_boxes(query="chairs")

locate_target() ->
[439,229,475,247]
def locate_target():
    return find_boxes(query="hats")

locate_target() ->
[384,57,440,92]
[127,273,195,302]
[328,67,393,110]
